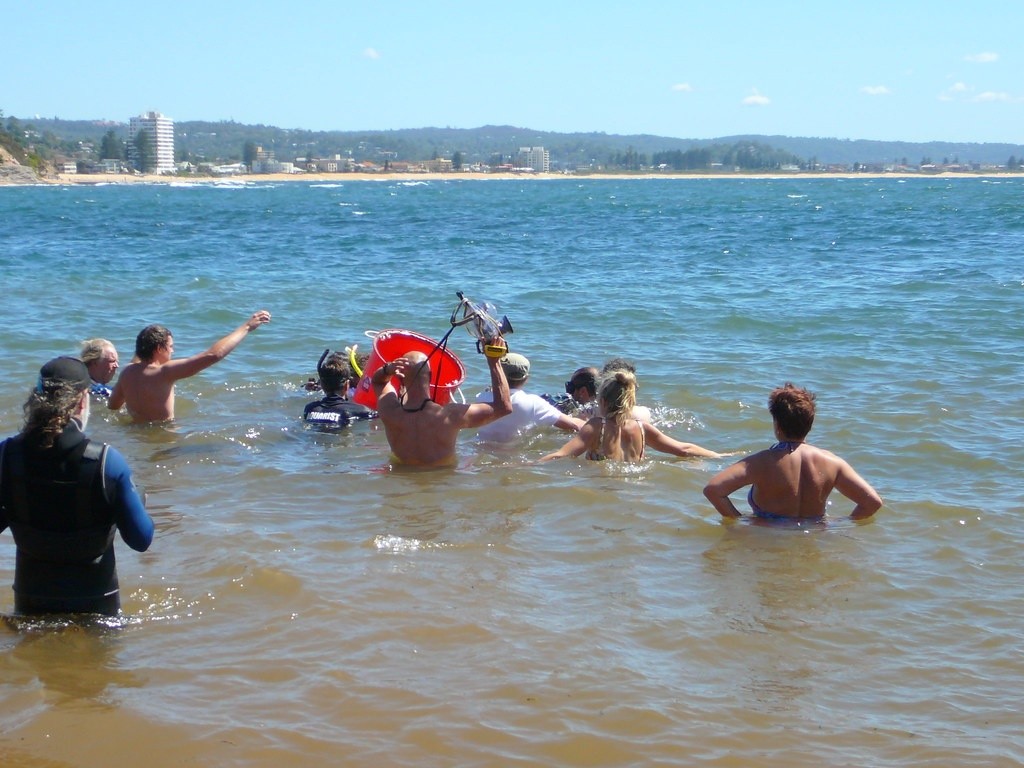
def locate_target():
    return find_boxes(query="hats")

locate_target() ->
[36,356,91,397]
[500,352,531,381]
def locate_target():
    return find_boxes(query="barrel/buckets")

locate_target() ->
[353,328,465,409]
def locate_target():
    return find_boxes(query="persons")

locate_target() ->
[303,357,378,422]
[372,336,513,457]
[703,383,882,517]
[107,310,271,419]
[81,338,120,398]
[542,370,695,459]
[565,368,599,404]
[575,359,651,425]
[0,357,155,614]
[476,353,588,433]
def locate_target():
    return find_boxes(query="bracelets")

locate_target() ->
[383,363,388,374]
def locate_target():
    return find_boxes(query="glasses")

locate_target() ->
[565,381,585,395]
